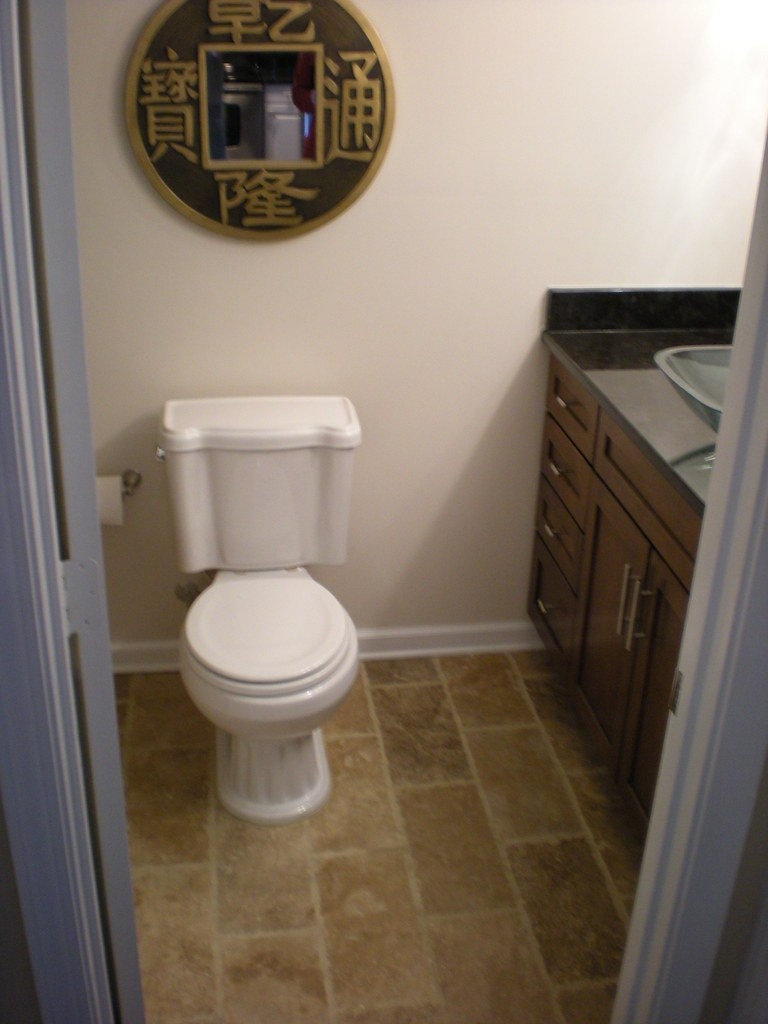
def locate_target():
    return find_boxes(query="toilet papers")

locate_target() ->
[96,474,123,527]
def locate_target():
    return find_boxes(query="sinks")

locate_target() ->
[652,343,735,434]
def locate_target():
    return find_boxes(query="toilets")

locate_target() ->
[156,393,372,824]
